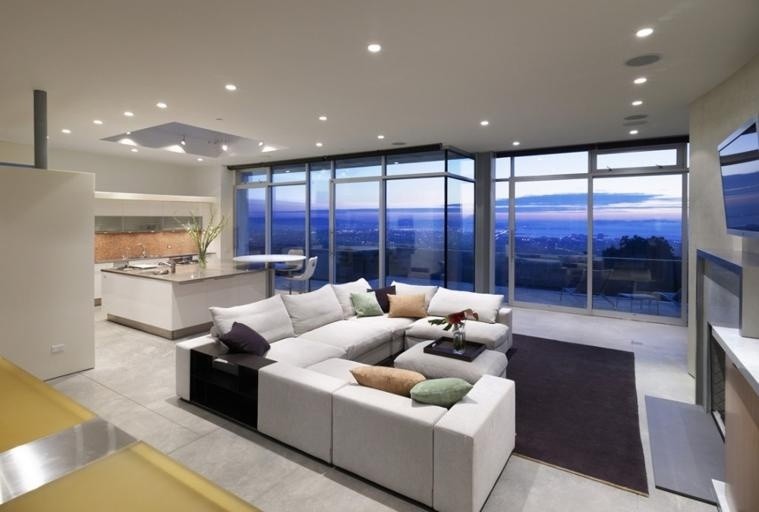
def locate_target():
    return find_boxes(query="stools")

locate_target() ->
[392,338,509,388]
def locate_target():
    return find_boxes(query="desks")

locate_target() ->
[232,254,306,299]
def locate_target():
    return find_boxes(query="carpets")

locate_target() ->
[373,324,651,501]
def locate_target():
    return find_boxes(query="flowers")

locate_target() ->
[430,310,480,332]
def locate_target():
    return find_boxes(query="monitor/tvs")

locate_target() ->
[717,119,759,238]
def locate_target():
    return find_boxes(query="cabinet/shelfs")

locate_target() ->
[192,253,217,262]
[163,202,205,232]
[129,258,170,267]
[94,198,122,233]
[93,262,113,299]
[704,325,759,512]
[121,199,163,233]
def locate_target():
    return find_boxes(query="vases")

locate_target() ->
[199,257,207,268]
[451,328,465,355]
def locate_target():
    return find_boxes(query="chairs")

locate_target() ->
[282,256,319,297]
[559,259,663,317]
[273,247,306,295]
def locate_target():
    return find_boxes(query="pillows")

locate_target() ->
[351,364,425,395]
[209,271,398,356]
[385,276,505,326]
[405,378,474,407]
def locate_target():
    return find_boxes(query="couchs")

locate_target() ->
[174,306,520,509]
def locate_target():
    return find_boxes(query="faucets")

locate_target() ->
[158,260,176,274]
